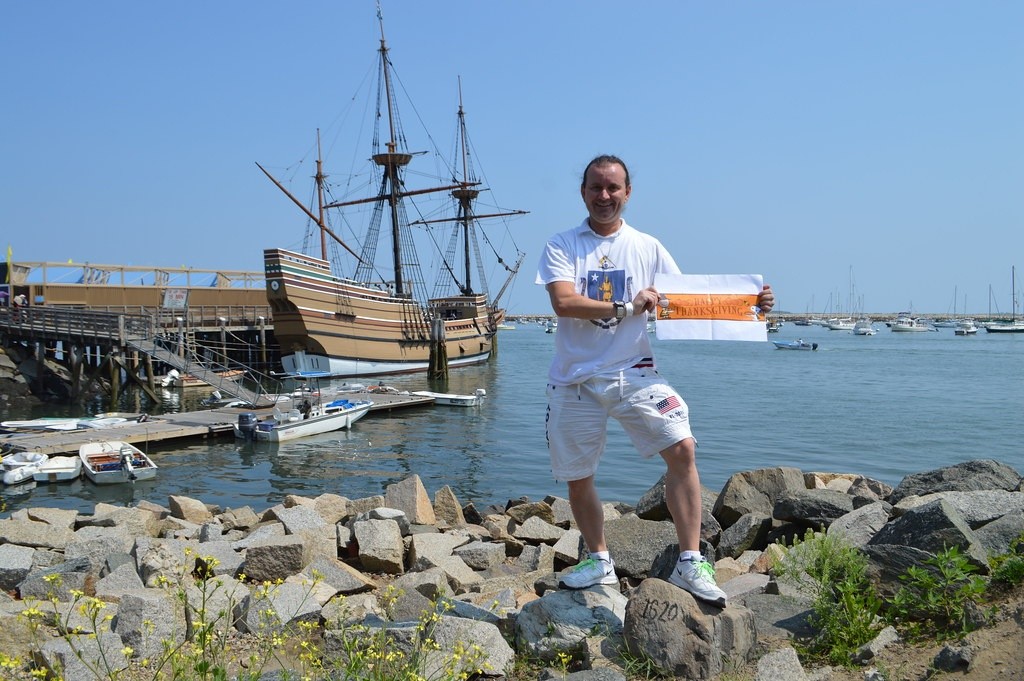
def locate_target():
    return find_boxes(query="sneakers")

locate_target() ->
[669,554,727,608]
[559,559,617,589]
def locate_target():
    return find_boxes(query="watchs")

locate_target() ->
[614,299,625,319]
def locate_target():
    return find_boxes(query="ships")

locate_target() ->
[892,312,929,331]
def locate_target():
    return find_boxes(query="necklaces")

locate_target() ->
[596,236,613,268]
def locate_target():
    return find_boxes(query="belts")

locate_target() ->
[633,357,655,368]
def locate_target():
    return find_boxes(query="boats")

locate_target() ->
[538,317,557,333]
[396,388,487,407]
[2,442,158,484]
[233,371,375,442]
[766,320,785,332]
[647,324,654,333]
[955,322,977,335]
[772,339,819,351]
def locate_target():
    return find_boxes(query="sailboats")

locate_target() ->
[794,265,879,335]
[977,265,1024,333]
[777,300,785,322]
[931,285,975,327]
[263,0,532,379]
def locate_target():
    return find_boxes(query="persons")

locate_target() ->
[334,276,394,302]
[535,155,774,609]
[797,338,802,345]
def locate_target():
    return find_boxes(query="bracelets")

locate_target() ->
[625,302,633,318]
